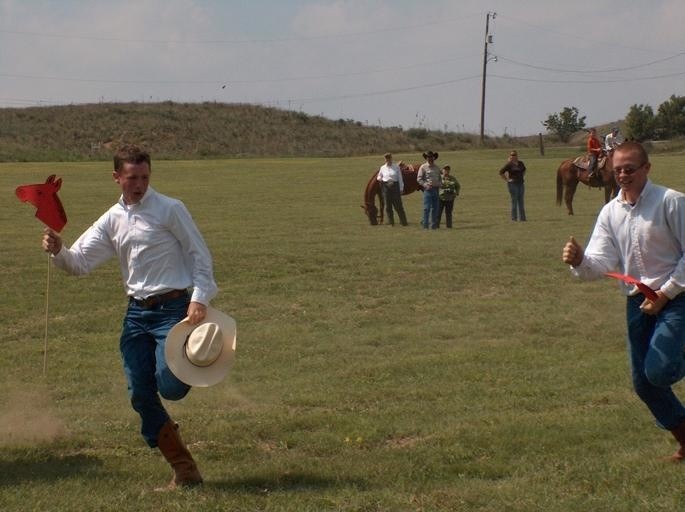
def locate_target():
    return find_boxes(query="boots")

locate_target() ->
[655,418,685,464]
[152,418,203,494]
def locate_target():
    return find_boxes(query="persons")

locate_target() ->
[41,145,219,492]
[437,165,460,229]
[377,153,408,228]
[417,150,442,231]
[586,128,603,177]
[499,151,526,223]
[604,129,621,151]
[562,142,685,461]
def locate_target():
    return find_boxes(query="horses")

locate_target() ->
[555,136,634,217]
[360,160,424,226]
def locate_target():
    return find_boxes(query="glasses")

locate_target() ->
[611,163,646,175]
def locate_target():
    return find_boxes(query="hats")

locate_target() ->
[384,153,391,157]
[611,127,620,132]
[443,163,451,170]
[422,150,438,161]
[165,306,235,389]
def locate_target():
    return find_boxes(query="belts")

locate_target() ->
[130,288,189,306]
